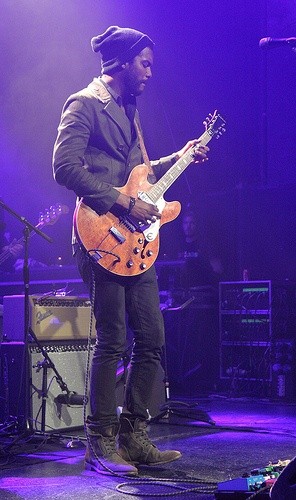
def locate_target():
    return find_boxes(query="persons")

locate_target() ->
[0,238,24,259]
[52,26,210,477]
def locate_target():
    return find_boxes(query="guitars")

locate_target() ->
[0,202,70,266]
[73,110,227,277]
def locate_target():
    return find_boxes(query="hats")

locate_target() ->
[91,26,154,74]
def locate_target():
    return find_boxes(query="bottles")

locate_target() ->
[276,369,286,398]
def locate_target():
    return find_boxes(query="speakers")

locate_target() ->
[0,343,96,432]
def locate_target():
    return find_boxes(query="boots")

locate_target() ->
[118,417,182,466]
[83,426,138,475]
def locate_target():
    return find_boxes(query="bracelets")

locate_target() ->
[128,197,135,213]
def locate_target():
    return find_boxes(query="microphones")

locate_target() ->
[259,37,296,48]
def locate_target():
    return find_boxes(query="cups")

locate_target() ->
[243,269,249,281]
